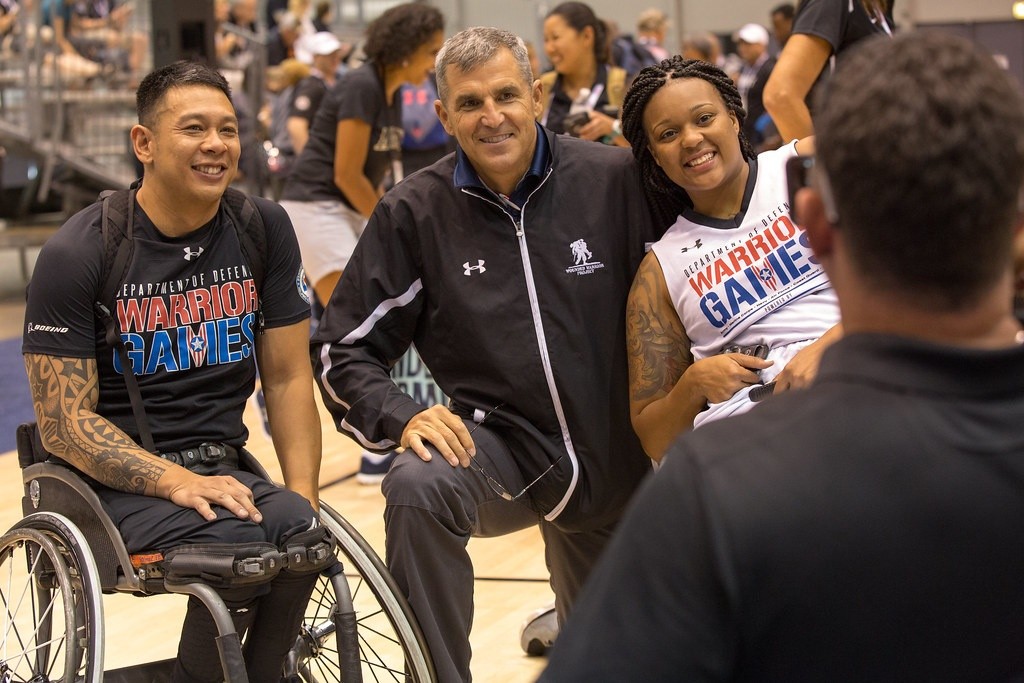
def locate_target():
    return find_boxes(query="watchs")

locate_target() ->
[601,119,623,144]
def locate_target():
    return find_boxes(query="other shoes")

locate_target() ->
[254,389,273,437]
[521,604,561,657]
[355,448,402,483]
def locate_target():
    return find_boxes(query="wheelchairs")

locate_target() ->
[0,417,440,682]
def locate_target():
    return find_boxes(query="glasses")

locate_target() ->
[461,405,564,504]
[785,150,840,227]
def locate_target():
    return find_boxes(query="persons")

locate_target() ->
[529,1,629,147]
[683,36,715,65]
[770,3,796,49]
[538,32,1024,682]
[269,30,343,196]
[762,1,898,145]
[278,1,446,487]
[310,25,660,682]
[605,21,658,78]
[21,63,341,682]
[620,54,843,464]
[709,30,743,81]
[731,23,779,125]
[637,8,672,61]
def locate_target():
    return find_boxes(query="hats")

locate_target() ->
[732,23,769,46]
[306,32,340,57]
